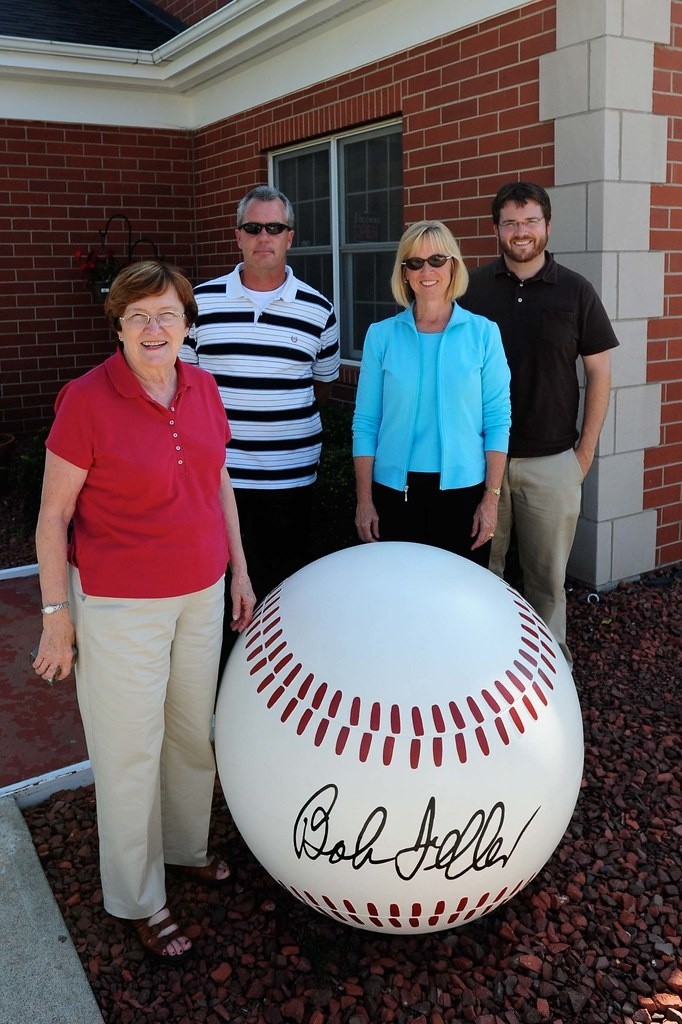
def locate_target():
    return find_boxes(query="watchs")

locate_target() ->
[484,486,501,496]
[41,601,69,615]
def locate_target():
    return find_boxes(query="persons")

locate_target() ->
[177,188,340,714]
[457,183,619,673]
[353,220,511,568]
[32,261,256,962]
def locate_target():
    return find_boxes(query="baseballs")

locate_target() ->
[213,537,591,942]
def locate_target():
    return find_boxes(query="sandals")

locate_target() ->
[174,857,230,884]
[131,900,191,955]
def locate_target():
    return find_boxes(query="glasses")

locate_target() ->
[402,254,453,271]
[498,216,545,231]
[119,312,186,327]
[238,223,292,235]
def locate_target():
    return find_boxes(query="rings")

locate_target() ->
[489,533,494,537]
